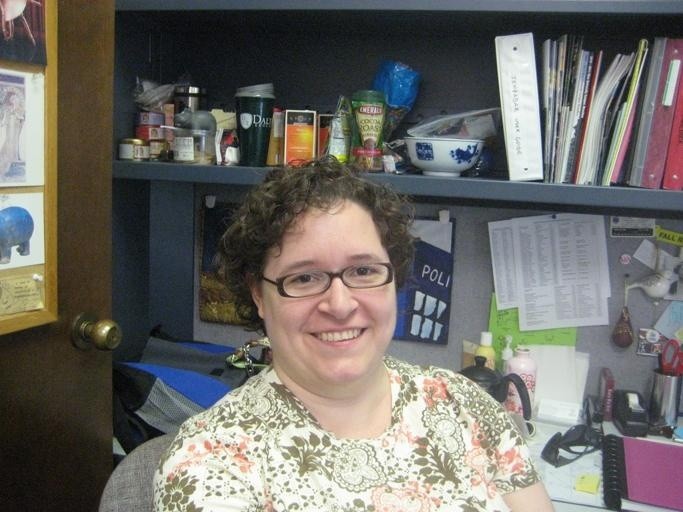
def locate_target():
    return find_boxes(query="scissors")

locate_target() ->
[662,340,683,377]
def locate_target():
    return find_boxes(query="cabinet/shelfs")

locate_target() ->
[114,1,681,512]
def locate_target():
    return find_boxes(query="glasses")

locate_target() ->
[260,263,394,298]
[541,425,602,468]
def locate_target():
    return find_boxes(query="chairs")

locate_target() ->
[100,433,177,512]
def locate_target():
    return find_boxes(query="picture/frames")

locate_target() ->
[1,0,57,336]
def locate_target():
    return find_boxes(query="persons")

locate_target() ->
[151,155,557,512]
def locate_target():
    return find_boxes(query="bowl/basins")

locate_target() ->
[405,137,486,178]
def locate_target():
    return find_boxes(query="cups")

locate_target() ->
[173,131,216,166]
[175,86,208,114]
[650,368,680,430]
[236,82,275,166]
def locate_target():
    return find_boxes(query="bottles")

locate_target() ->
[504,349,536,420]
[474,332,496,371]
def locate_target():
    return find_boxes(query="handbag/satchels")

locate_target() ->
[112,325,258,455]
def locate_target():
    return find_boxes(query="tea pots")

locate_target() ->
[459,357,531,420]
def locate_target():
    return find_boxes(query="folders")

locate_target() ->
[495,32,545,182]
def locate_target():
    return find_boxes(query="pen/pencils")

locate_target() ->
[657,352,663,374]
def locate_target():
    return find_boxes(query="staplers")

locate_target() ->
[582,395,605,436]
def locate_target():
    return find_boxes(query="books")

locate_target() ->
[602,433,683,512]
[542,34,682,192]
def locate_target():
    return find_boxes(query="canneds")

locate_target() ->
[118,138,151,163]
[148,136,166,160]
[134,110,164,128]
[135,124,160,142]
